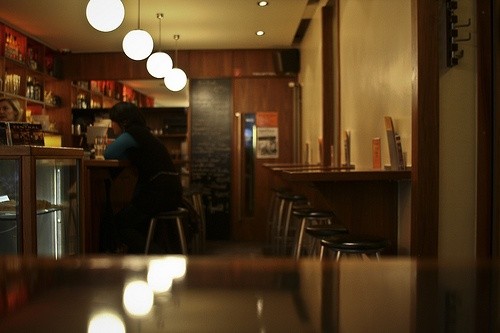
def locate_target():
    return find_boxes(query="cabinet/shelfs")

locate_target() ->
[71,79,156,107]
[0,20,55,107]
[72,108,191,159]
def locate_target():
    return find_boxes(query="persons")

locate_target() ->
[98,101,182,253]
[0,97,23,121]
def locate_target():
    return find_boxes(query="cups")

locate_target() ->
[30,115,49,124]
[43,130,61,147]
[4,74,20,96]
[87,126,108,144]
[94,135,106,161]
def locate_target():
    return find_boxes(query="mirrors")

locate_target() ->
[70,80,189,108]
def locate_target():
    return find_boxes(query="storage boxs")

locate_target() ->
[87,126,113,145]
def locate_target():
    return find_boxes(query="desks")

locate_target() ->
[263,162,408,183]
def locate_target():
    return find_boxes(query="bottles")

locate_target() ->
[4,32,22,61]
[26,75,41,101]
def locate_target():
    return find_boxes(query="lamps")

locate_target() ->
[164,35,187,91]
[122,0,154,60]
[147,13,172,78]
[86,0,124,32]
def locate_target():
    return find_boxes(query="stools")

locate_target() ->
[145,209,192,257]
[271,187,385,259]
